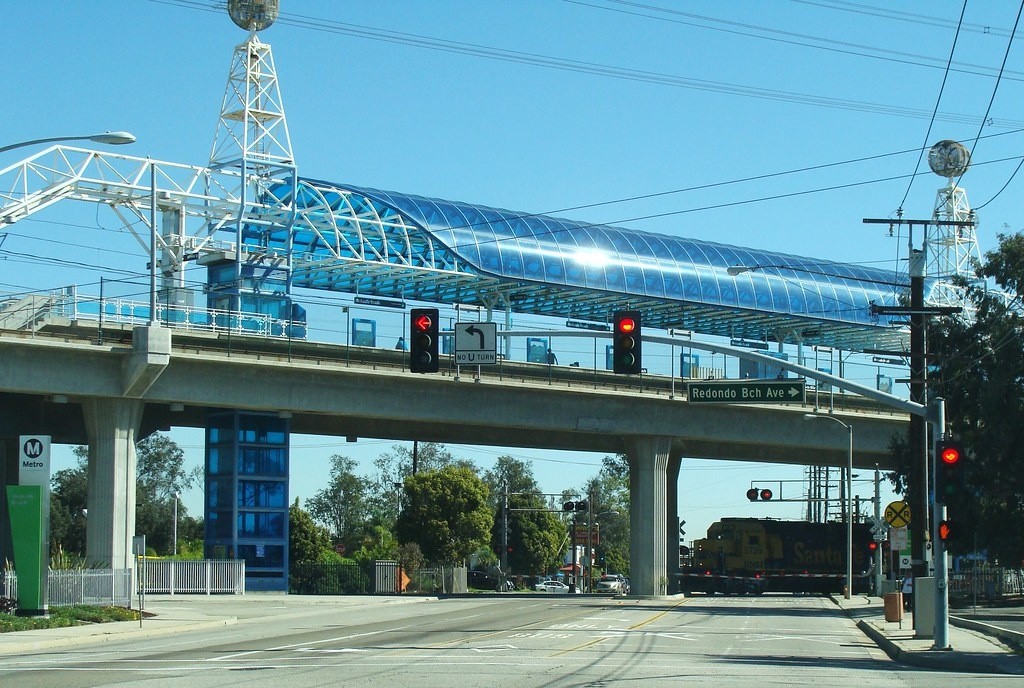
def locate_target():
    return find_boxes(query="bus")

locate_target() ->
[951,548,1003,603]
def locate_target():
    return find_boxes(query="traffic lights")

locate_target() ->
[613,310,642,374]
[933,439,964,504]
[566,501,586,511]
[747,488,772,501]
[865,524,877,552]
[937,520,954,542]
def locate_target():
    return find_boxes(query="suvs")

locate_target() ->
[467,571,516,591]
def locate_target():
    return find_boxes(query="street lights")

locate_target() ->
[803,413,853,600]
[728,263,928,631]
[170,491,179,555]
[588,511,619,592]
[393,483,404,546]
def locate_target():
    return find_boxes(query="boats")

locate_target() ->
[410,308,439,372]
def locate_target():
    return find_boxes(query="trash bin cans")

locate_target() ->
[885,593,904,623]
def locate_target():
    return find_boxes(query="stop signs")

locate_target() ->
[335,544,346,556]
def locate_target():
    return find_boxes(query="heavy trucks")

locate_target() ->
[677,517,871,595]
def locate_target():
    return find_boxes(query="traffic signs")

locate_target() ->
[455,322,496,364]
[687,379,806,405]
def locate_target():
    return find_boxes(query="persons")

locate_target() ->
[547,348,558,365]
[902,570,912,612]
[396,337,408,351]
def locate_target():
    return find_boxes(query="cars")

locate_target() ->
[596,573,630,594]
[534,580,581,594]
